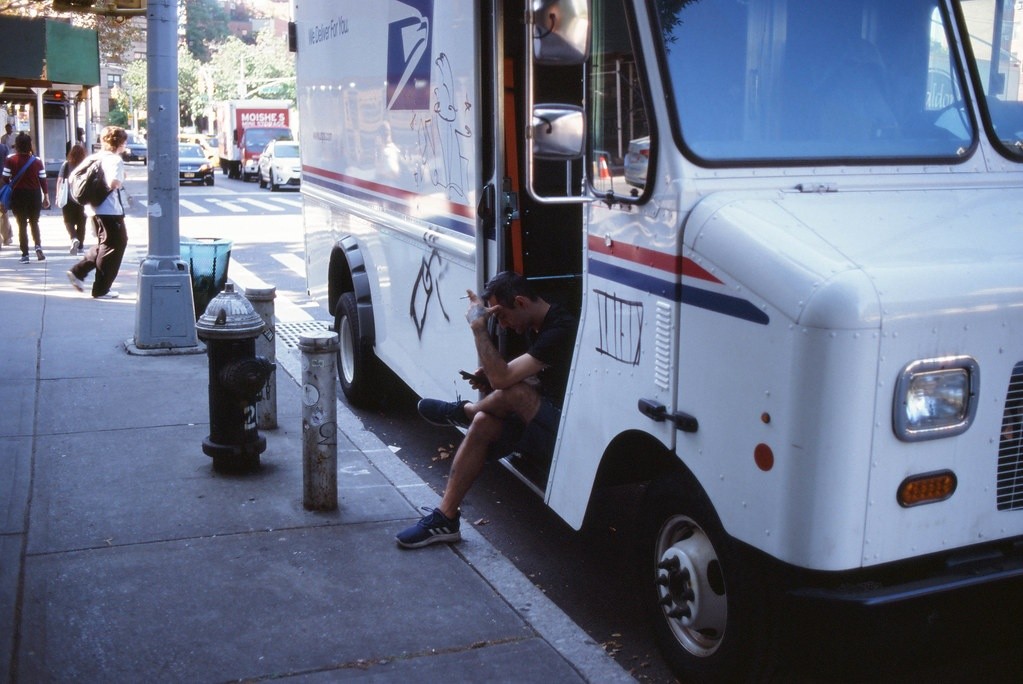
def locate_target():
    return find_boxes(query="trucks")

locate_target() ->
[217,98,291,181]
[288,0,1022,684]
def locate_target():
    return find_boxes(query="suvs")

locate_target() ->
[258,141,301,192]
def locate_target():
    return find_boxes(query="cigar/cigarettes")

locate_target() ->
[459,294,471,299]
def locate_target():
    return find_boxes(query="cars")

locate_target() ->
[177,142,215,185]
[179,134,219,167]
[118,132,147,165]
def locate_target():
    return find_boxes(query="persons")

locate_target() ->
[2,124,17,154]
[67,127,87,158]
[392,269,579,549]
[0,144,14,249]
[2,130,51,265]
[55,144,88,256]
[66,126,129,299]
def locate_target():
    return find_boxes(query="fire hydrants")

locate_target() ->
[195,283,276,476]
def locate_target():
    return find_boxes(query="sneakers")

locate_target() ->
[92,289,120,300]
[69,238,80,256]
[67,270,85,292]
[76,249,84,253]
[35,245,45,260]
[417,397,474,430]
[18,253,31,264]
[394,506,461,548]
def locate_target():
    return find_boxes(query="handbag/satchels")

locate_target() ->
[56,161,68,209]
[0,184,13,211]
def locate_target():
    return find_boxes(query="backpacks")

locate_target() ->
[68,151,114,207]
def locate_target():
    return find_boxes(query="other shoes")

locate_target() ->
[0,237,12,251]
